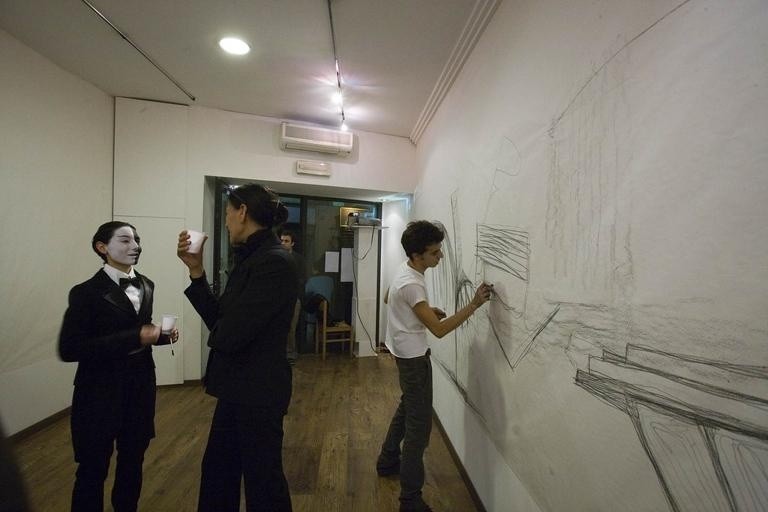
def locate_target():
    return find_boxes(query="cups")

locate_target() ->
[160,312,179,336]
[184,230,206,254]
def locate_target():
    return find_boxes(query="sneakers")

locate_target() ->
[376,453,403,475]
[399,499,432,512]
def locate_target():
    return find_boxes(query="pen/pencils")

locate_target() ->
[169,337,174,356]
[480,284,494,289]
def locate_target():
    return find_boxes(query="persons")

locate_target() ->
[375,220,494,512]
[280,230,306,365]
[176,182,293,512]
[57,220,179,512]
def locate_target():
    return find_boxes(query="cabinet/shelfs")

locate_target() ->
[341,225,381,358]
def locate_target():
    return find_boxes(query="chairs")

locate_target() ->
[314,299,354,360]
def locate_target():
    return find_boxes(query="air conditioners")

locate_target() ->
[279,121,354,155]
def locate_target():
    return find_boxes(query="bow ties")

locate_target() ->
[119,277,141,291]
